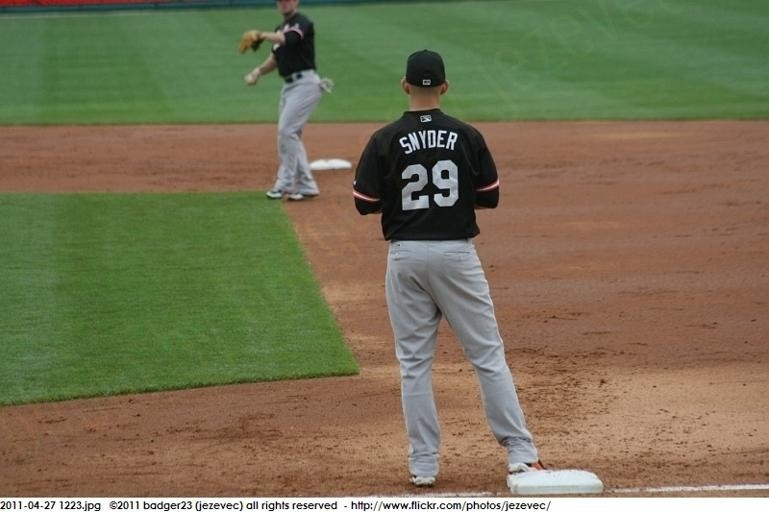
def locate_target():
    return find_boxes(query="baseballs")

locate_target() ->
[246,75,256,85]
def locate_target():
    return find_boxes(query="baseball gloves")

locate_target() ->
[238,29,264,55]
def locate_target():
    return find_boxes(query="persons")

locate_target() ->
[237,0,334,202]
[351,49,547,487]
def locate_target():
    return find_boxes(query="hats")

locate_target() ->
[404,49,446,89]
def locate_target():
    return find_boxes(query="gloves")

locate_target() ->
[243,67,261,86]
[317,77,335,95]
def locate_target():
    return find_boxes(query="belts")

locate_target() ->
[282,73,304,85]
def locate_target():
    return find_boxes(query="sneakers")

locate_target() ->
[409,464,441,487]
[506,447,547,474]
[266,185,293,200]
[287,186,320,202]
